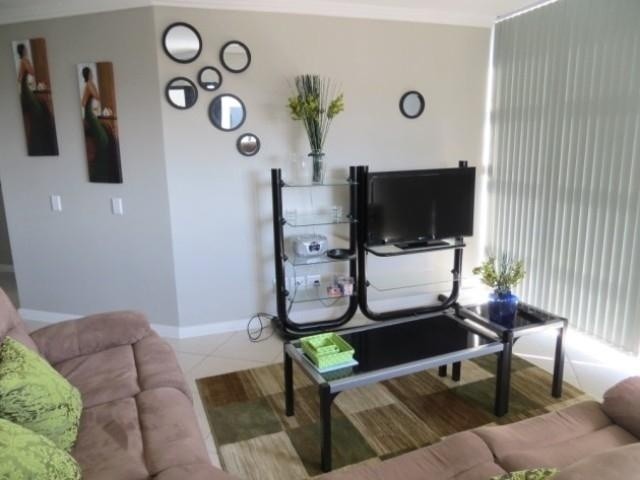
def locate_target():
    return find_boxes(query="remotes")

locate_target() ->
[518,307,545,322]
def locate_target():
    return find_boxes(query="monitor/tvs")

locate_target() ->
[366,166,476,249]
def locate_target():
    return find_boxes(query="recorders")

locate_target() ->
[293,235,328,258]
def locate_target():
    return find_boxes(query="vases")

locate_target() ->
[489,285,521,330]
[309,149,328,183]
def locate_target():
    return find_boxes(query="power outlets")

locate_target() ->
[306,273,323,290]
[290,275,306,292]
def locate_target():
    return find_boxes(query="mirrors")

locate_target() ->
[398,90,425,118]
[158,22,262,156]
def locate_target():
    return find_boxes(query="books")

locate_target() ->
[295,348,360,375]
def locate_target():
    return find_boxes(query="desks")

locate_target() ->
[279,312,509,472]
[451,298,569,418]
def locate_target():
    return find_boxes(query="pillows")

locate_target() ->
[0,418,83,480]
[0,332,85,449]
[487,466,563,479]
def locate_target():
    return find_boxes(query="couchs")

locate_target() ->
[305,371,640,479]
[0,285,208,480]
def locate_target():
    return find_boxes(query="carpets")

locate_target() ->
[192,339,597,479]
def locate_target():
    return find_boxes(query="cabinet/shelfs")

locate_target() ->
[270,165,359,333]
[359,160,473,323]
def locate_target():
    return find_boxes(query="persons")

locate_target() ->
[81,65,111,177]
[17,43,44,153]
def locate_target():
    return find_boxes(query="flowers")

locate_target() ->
[287,72,347,177]
[471,250,527,296]
